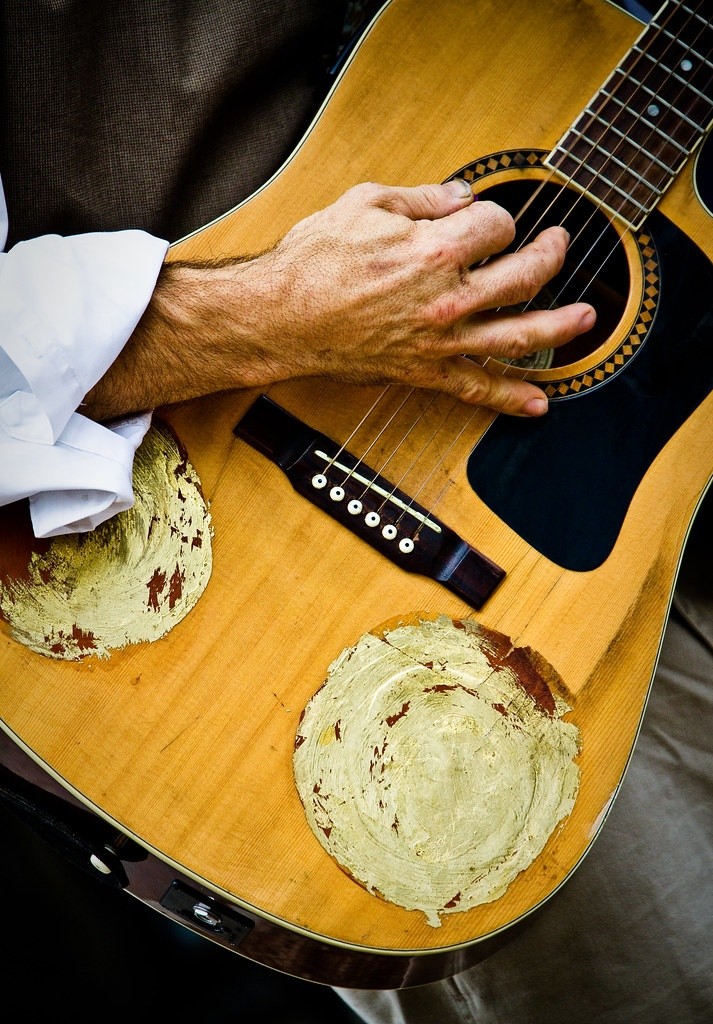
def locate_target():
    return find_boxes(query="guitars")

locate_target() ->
[0,0,713,991]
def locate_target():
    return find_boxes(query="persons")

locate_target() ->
[1,0,713,1024]
